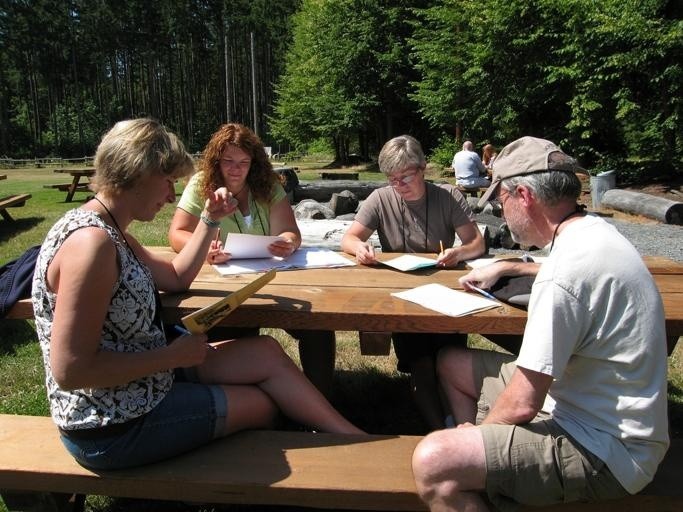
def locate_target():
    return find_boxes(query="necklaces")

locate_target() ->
[232,184,248,198]
[400,180,433,254]
[549,205,586,252]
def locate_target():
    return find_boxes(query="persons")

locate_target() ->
[451,140,498,198]
[168,123,302,264]
[342,135,486,433]
[32,118,368,471]
[412,135,669,512]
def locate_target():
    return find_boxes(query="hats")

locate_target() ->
[477,136,577,208]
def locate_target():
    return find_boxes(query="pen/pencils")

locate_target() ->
[187,331,218,353]
[210,228,220,266]
[466,282,496,300]
[440,240,446,267]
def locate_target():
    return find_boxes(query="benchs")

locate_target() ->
[455,184,489,197]
[43,182,94,192]
[0,413,683,512]
[0,193,32,225]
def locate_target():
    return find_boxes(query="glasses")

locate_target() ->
[388,169,418,188]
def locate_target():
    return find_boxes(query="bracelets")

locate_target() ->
[200,214,221,228]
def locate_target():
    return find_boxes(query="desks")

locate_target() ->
[3,245,683,356]
[0,173,8,180]
[443,167,588,183]
[54,168,97,203]
